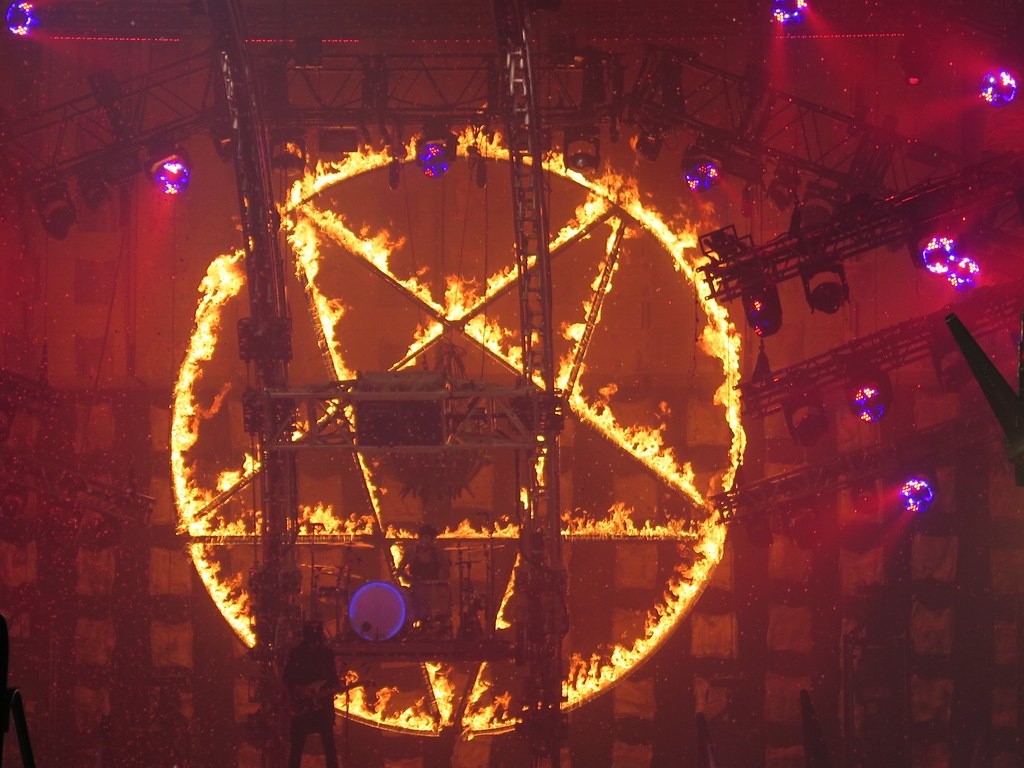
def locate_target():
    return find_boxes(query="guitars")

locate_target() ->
[281,677,373,718]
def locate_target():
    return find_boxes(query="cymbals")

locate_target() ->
[318,565,362,581]
[441,545,479,552]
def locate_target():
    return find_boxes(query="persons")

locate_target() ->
[396,523,452,641]
[279,620,340,768]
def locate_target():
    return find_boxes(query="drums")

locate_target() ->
[346,581,406,644]
[418,579,450,605]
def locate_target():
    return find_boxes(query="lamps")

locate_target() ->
[905,225,960,277]
[975,60,1016,111]
[896,470,941,513]
[770,0,809,26]
[847,366,896,425]
[0,0,42,36]
[144,137,194,201]
[413,121,460,181]
[678,141,723,193]
[944,251,981,291]
